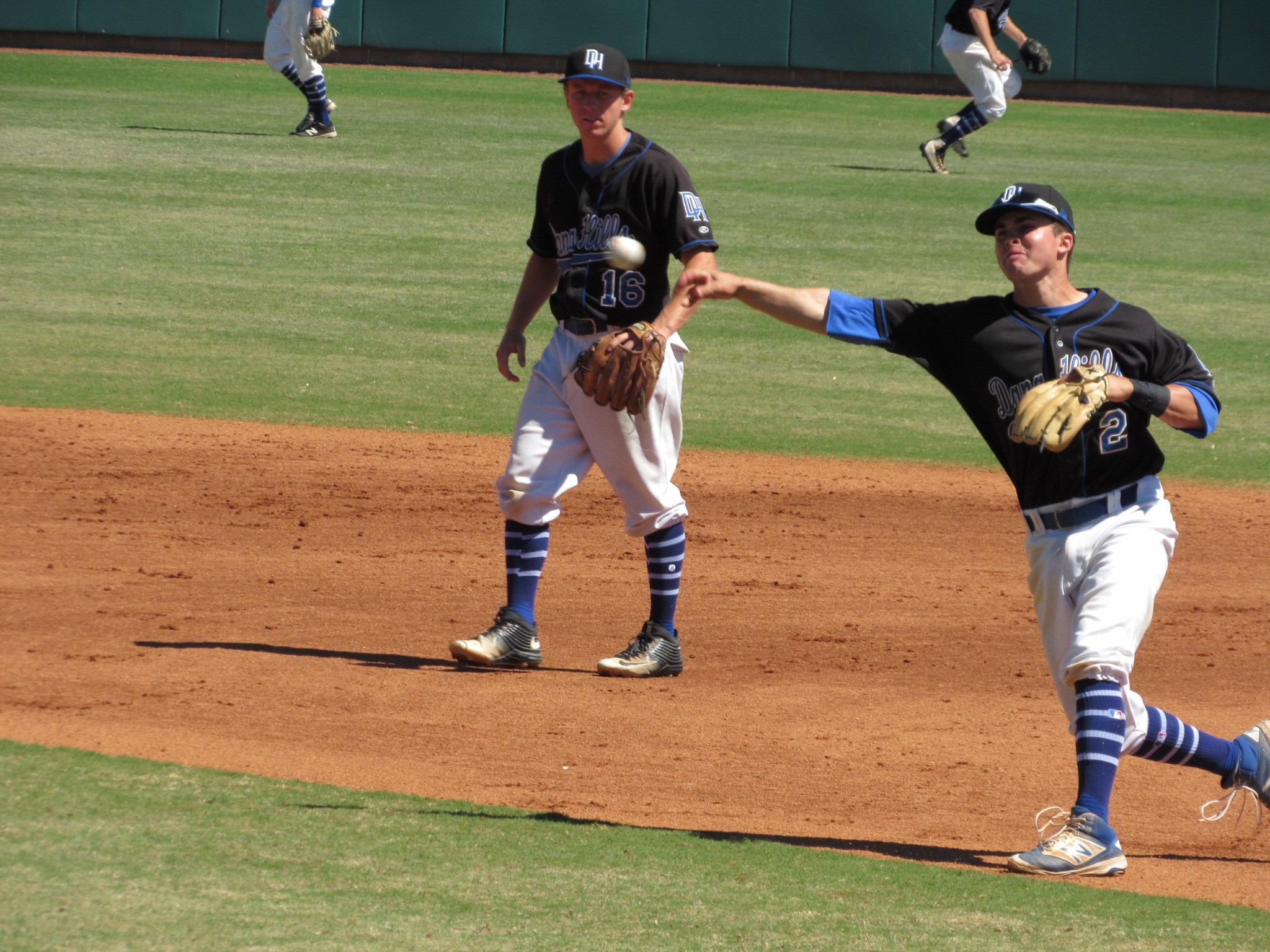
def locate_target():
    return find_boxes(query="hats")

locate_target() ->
[975,183,1076,237]
[557,43,631,89]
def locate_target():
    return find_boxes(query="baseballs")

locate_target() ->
[604,235,647,272]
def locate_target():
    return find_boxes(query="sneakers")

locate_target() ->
[936,115,969,158]
[597,620,683,677]
[919,139,949,173]
[289,120,337,137]
[1008,806,1128,877]
[296,98,337,132]
[448,606,542,668]
[1198,719,1270,826]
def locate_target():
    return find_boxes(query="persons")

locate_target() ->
[676,182,1270,878]
[918,1,1055,176]
[451,44,722,679]
[264,0,341,139]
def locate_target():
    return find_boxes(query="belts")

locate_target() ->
[1024,484,1139,531]
[558,316,607,336]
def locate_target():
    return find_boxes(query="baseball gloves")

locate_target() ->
[302,18,338,60]
[568,320,667,414]
[1010,362,1110,453]
[1018,38,1052,75]
